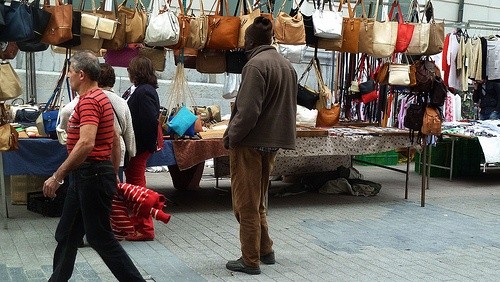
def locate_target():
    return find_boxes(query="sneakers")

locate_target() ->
[226,257,261,275]
[261,250,275,264]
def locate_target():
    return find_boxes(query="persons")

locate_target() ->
[222,16,299,275]
[122,56,160,241]
[42,51,146,282]
[473,80,500,119]
[55,63,136,247]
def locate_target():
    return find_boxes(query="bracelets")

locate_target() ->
[53,174,64,185]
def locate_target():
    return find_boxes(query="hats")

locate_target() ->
[245,16,273,45]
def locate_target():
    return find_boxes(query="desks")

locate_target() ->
[0,119,500,231]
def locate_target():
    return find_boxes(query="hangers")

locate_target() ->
[401,91,415,98]
[451,23,500,45]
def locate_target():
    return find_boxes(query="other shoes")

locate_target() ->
[124,232,155,241]
[77,240,84,249]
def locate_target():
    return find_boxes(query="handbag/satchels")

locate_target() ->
[0,0,447,151]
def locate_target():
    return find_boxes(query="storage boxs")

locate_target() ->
[354,150,399,165]
[414,138,484,179]
[10,175,42,205]
